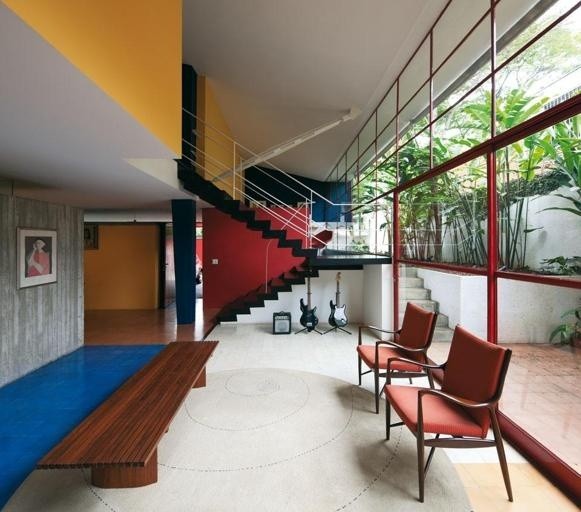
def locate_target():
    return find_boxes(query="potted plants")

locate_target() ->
[549,307,581,348]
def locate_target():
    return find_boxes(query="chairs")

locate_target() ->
[356,302,513,503]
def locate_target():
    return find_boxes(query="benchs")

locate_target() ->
[35,340,221,488]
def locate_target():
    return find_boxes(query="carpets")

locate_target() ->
[2,368,473,512]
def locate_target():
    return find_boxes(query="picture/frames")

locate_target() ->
[16,226,60,290]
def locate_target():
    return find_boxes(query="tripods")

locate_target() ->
[295,292,323,335]
[323,292,352,334]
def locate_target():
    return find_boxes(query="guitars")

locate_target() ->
[328,272,347,328]
[300,268,318,330]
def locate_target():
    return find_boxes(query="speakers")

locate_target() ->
[273,312,291,334]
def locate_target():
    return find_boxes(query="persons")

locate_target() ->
[27,237,51,279]
